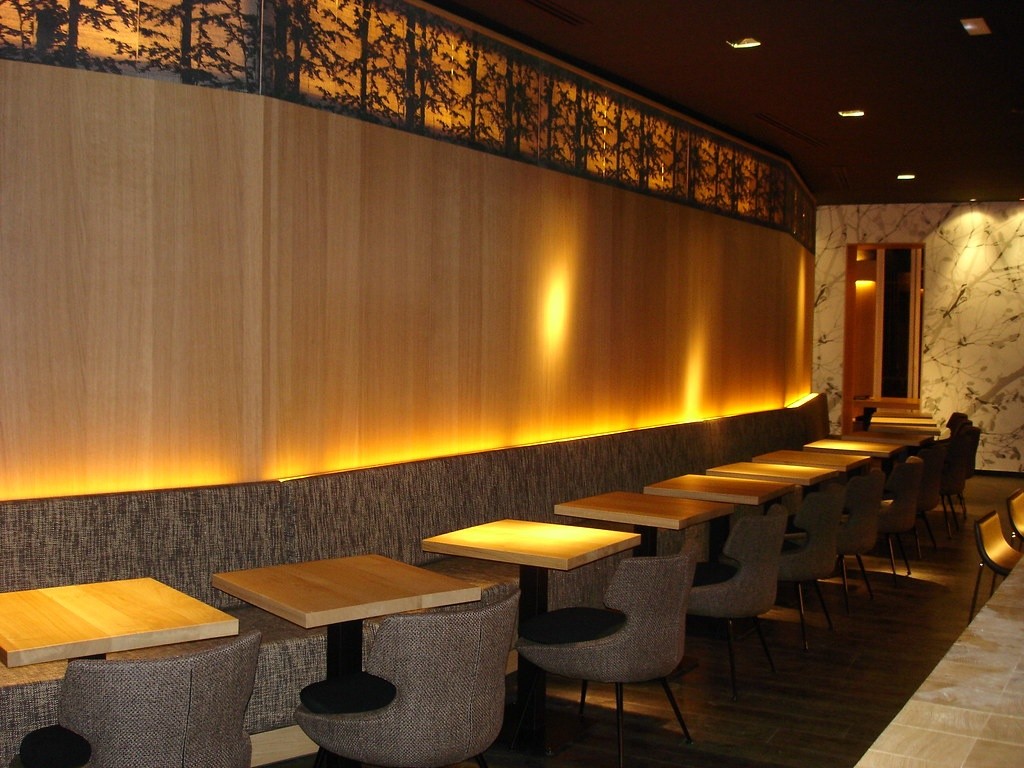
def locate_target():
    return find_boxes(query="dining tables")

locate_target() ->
[420,516,672,768]
[752,449,871,484]
[865,408,941,437]
[553,492,737,687]
[643,473,798,557]
[211,553,485,676]
[802,438,905,475]
[0,576,239,672]
[840,430,934,459]
[705,462,841,515]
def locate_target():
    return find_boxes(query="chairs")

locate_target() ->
[775,484,852,650]
[966,507,1024,628]
[514,556,709,768]
[4,629,263,768]
[1006,485,1024,551]
[929,409,981,541]
[876,454,926,585]
[677,499,791,702]
[289,588,522,768]
[837,465,886,609]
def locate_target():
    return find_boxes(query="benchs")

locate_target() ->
[0,392,836,768]
